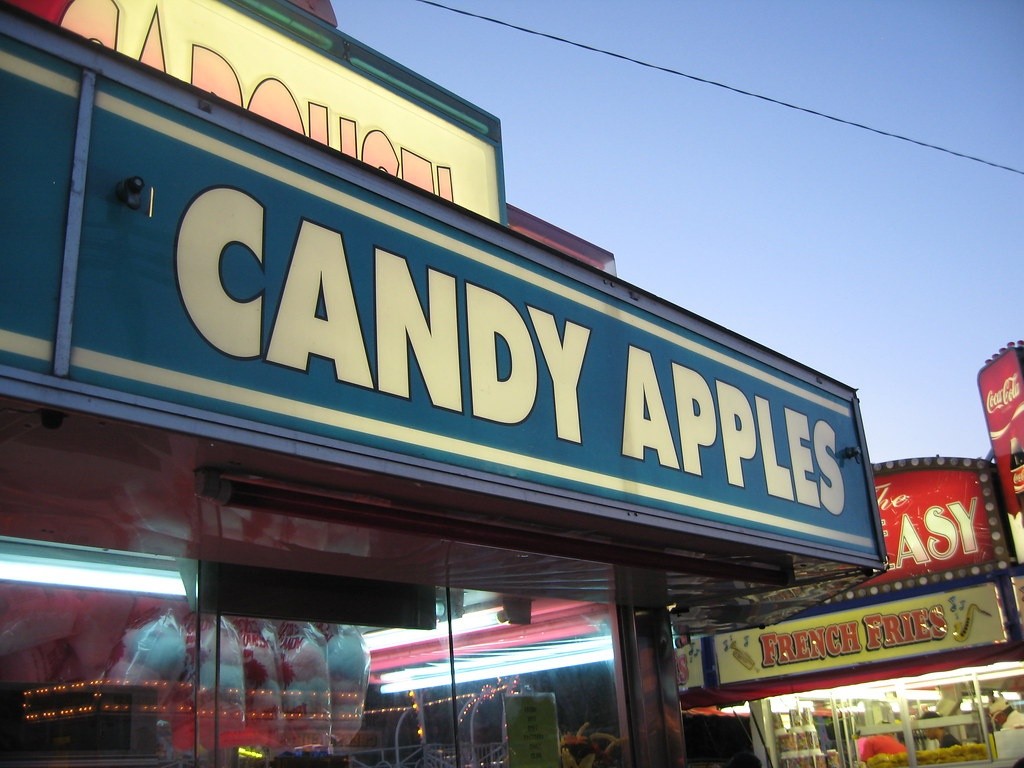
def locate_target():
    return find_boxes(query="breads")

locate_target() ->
[867,743,988,768]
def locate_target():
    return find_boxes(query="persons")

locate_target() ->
[988,697,1024,730]
[859,720,908,762]
[919,711,962,747]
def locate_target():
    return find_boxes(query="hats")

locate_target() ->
[988,697,1010,715]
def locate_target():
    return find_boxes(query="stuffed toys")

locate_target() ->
[95,600,371,747]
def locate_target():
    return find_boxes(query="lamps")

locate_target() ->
[361,597,532,651]
[0,541,466,619]
[188,465,798,591]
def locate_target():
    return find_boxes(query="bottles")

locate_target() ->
[1010,435,1024,528]
